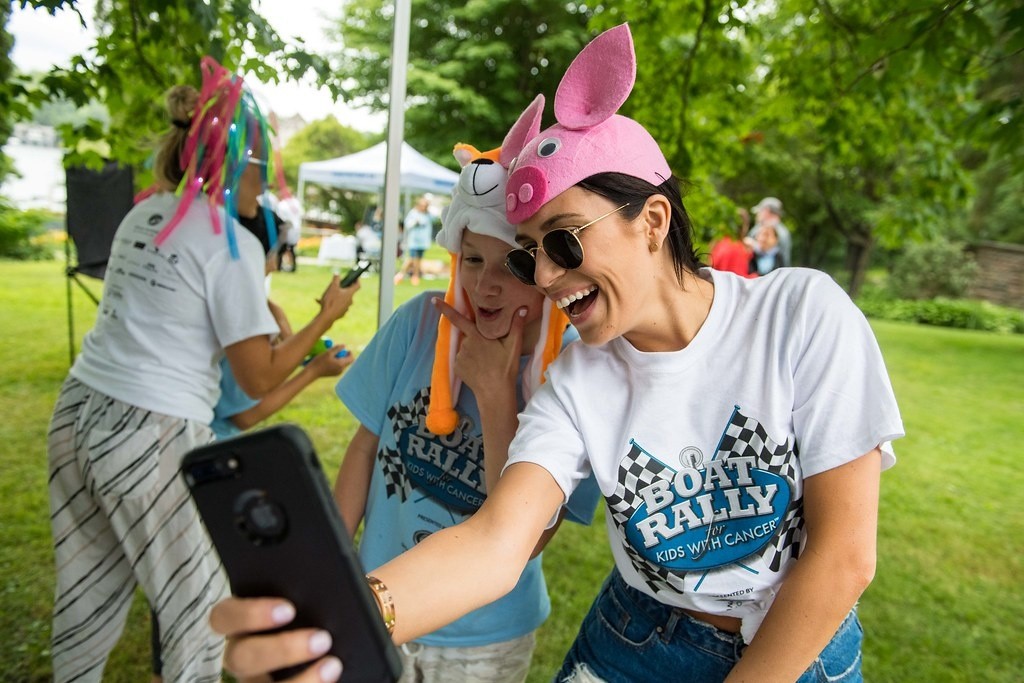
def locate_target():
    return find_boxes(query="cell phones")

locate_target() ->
[181,423,404,683]
[339,260,371,289]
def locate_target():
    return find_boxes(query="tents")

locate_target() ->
[297,140,460,253]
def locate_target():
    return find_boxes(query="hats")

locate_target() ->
[749,197,784,215]
[497,21,672,224]
[425,143,571,435]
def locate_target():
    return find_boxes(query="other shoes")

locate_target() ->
[394,272,403,285]
[411,275,419,285]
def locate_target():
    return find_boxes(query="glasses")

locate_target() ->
[505,203,629,287]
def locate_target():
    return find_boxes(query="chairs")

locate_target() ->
[66,164,138,367]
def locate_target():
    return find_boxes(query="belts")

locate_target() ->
[682,608,742,636]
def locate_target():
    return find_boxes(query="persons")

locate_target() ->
[48,56,362,683]
[395,198,432,285]
[708,197,792,280]
[355,222,381,260]
[211,22,904,683]
[398,223,404,256]
[151,206,355,683]
[237,187,301,296]
[331,143,600,683]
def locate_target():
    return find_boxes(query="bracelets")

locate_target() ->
[365,575,395,638]
